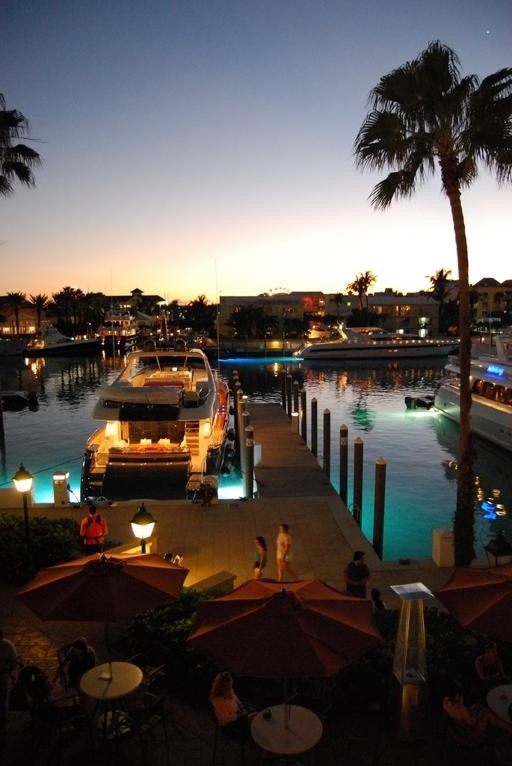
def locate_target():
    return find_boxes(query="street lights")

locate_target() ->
[395,327,404,341]
[128,502,157,554]
[418,316,429,338]
[10,463,37,577]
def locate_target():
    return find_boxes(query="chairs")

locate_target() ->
[14,633,183,764]
[208,671,285,766]
[440,697,488,766]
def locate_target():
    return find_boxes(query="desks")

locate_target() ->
[250,704,324,766]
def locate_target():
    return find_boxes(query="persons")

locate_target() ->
[442,686,512,738]
[474,641,505,693]
[209,671,258,745]
[253,537,267,579]
[343,551,369,598]
[276,525,299,582]
[164,553,173,562]
[0,631,96,722]
[172,552,185,568]
[371,589,387,612]
[79,505,108,556]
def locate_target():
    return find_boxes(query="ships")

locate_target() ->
[299,327,461,358]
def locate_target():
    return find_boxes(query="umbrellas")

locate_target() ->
[436,564,512,642]
[16,552,189,679]
[186,579,383,729]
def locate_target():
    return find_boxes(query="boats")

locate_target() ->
[22,320,100,352]
[80,337,236,502]
[433,333,512,453]
